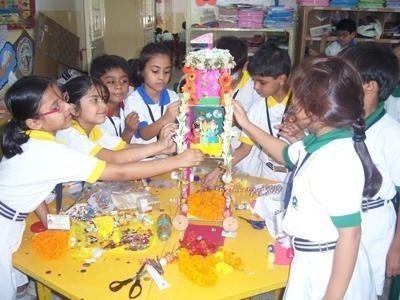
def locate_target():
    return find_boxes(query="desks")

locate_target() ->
[11,172,291,300]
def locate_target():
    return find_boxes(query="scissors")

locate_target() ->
[238,216,265,230]
[110,263,147,298]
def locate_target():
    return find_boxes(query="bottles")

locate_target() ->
[157,213,171,241]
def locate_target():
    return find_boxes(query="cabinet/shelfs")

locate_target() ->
[295,6,400,68]
[184,27,294,72]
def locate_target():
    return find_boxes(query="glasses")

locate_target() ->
[34,91,70,118]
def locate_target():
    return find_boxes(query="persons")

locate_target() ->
[307,18,358,60]
[1,73,204,300]
[233,56,383,300]
[90,53,141,146]
[56,75,178,164]
[230,43,295,183]
[383,44,400,124]
[123,42,182,142]
[273,39,399,300]
[214,35,263,179]
